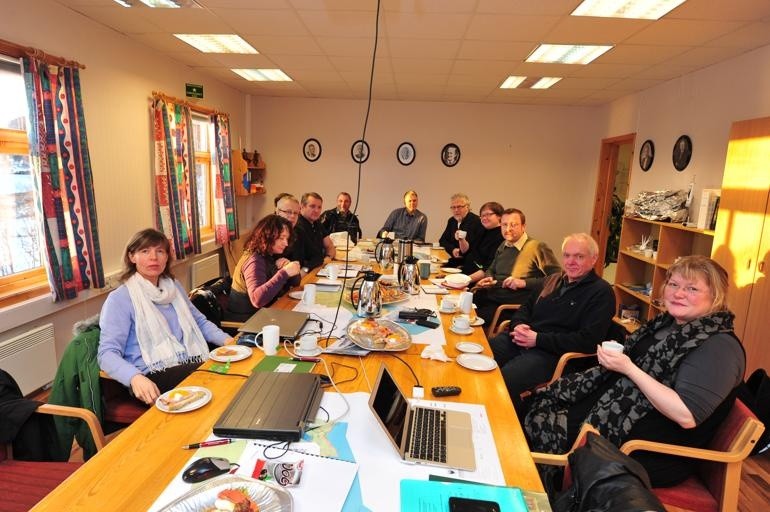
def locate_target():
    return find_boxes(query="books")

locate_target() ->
[709,196,720,228]
[696,189,720,229]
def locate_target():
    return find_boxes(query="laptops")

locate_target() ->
[214,371,325,442]
[367,361,477,472]
[237,308,310,341]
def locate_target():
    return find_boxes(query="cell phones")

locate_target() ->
[448,496,501,512]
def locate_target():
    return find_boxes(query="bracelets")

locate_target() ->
[301,266,309,272]
[325,254,335,260]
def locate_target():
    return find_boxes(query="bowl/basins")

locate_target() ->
[444,273,471,288]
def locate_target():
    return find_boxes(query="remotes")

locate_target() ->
[432,386,461,397]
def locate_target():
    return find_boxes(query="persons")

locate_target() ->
[438,193,481,268]
[443,146,458,165]
[641,145,651,170]
[376,191,428,245]
[401,147,410,160]
[320,192,362,243]
[487,232,616,398]
[274,192,295,215]
[673,138,690,169]
[306,144,316,158]
[230,215,301,322]
[470,208,563,327]
[454,202,505,282]
[274,196,321,279]
[518,255,745,489]
[96,228,234,408]
[293,192,336,271]
[355,144,365,158]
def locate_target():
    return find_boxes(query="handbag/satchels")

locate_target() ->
[555,430,667,512]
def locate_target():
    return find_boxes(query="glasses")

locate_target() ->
[279,209,301,217]
[480,212,495,217]
[449,204,465,210]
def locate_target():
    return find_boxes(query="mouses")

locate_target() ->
[237,334,263,346]
[182,456,230,483]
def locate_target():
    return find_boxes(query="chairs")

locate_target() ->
[490,320,624,405]
[471,274,561,336]
[46,314,222,442]
[186,277,289,337]
[530,390,769,512]
[1,368,109,510]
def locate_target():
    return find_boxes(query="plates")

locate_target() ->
[293,347,323,357]
[288,289,306,300]
[442,268,463,273]
[455,315,484,326]
[455,353,497,371]
[456,341,484,354]
[449,326,474,335]
[209,345,252,362]
[155,386,212,414]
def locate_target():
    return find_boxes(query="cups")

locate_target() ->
[326,264,338,280]
[460,292,473,313]
[459,231,467,239]
[440,299,457,313]
[645,248,653,257]
[652,252,657,259]
[452,316,469,330]
[294,335,317,351]
[418,262,431,280]
[301,283,316,306]
[602,342,623,354]
[254,324,280,357]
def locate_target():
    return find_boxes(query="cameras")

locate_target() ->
[415,318,440,329]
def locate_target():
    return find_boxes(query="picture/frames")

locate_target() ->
[672,134,692,171]
[303,138,322,161]
[396,141,415,166]
[639,139,655,171]
[351,140,369,163]
[440,142,461,167]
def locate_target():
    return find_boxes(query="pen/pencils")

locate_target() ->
[289,357,319,363]
[185,438,235,451]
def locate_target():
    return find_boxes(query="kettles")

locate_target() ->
[398,237,414,265]
[350,270,382,318]
[397,255,422,295]
[374,237,395,270]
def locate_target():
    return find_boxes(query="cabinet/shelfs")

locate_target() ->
[612,208,714,340]
[232,149,267,197]
[710,115,770,381]
